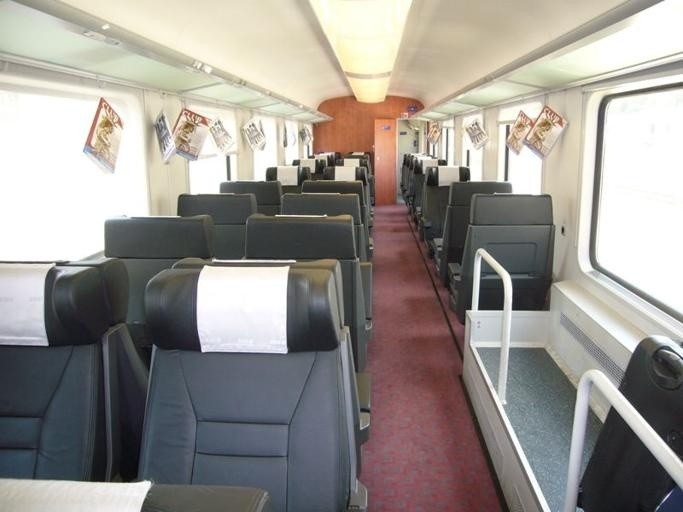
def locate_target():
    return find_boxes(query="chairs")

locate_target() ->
[428,182,513,287]
[314,151,341,166]
[407,157,438,219]
[334,157,376,207]
[308,155,332,169]
[401,153,428,194]
[176,191,257,266]
[402,154,432,204]
[141,268,371,512]
[101,214,216,354]
[445,194,558,325]
[221,182,283,216]
[277,192,373,267]
[341,153,375,203]
[303,178,375,261]
[412,160,448,224]
[175,258,372,479]
[0,265,118,484]
[398,154,407,192]
[264,167,309,196]
[245,215,373,371]
[4,256,148,483]
[415,167,471,243]
[292,159,326,182]
[347,151,372,176]
[323,167,374,229]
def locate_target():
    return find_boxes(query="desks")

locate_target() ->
[0,478,268,512]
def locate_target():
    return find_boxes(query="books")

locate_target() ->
[154,109,177,165]
[241,116,265,151]
[523,105,568,159]
[464,119,489,151]
[300,126,311,145]
[505,110,534,156]
[428,124,441,145]
[259,120,266,150]
[283,123,287,147]
[172,109,211,160]
[209,118,234,152]
[83,97,124,174]
